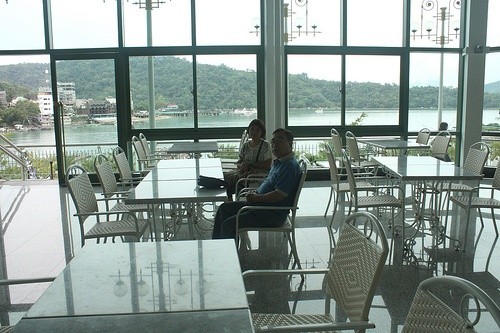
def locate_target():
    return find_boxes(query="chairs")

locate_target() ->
[65,128,500,333]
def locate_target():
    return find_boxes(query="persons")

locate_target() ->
[213,128,302,251]
[224,119,272,202]
[433,122,452,162]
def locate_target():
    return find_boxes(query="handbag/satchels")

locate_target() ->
[198,176,228,189]
[241,166,266,178]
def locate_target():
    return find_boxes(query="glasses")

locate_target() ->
[269,139,288,146]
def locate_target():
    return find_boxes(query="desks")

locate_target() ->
[168,146,218,159]
[124,178,229,241]
[370,156,453,235]
[22,239,249,319]
[369,142,430,196]
[142,166,224,235]
[154,158,221,219]
[383,164,482,266]
